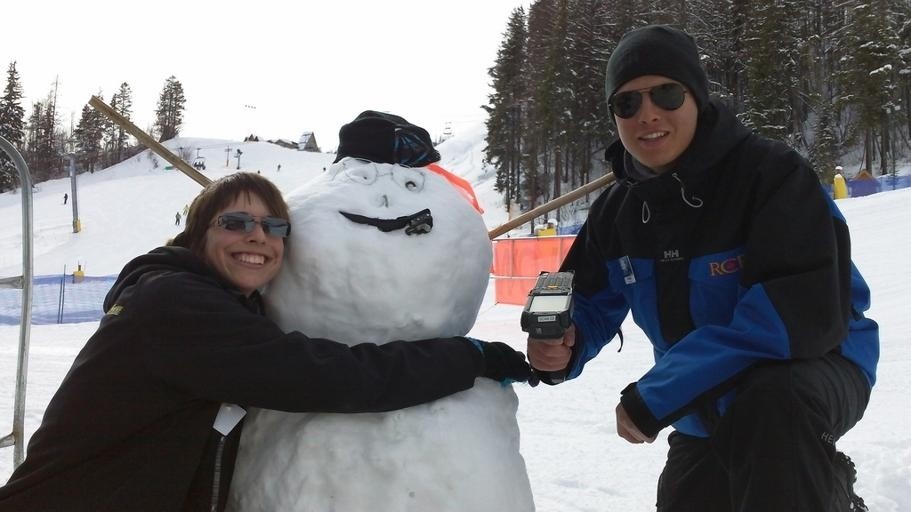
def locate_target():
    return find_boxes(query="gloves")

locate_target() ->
[470,337,532,385]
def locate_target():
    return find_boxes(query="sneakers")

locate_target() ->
[826,451,870,512]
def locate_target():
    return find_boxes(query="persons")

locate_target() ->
[63,193,68,204]
[526,22,881,510]
[174,212,181,226]
[829,165,849,199]
[0,171,534,510]
[546,217,559,230]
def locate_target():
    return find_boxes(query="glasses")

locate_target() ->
[332,158,427,192]
[610,83,691,122]
[208,209,289,243]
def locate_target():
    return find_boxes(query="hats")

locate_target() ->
[603,24,710,111]
[326,106,443,172]
